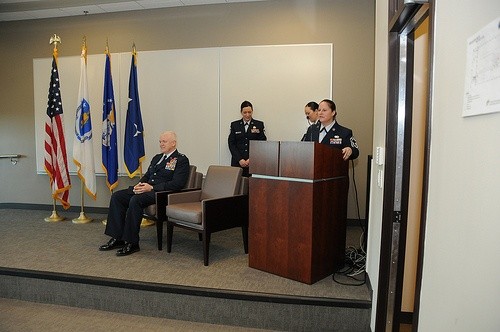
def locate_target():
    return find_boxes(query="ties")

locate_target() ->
[155,155,167,168]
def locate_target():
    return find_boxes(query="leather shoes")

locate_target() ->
[116,240,140,257]
[99,237,126,251]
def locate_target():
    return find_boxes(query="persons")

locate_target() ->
[304,99,360,160]
[228,100,267,177]
[305,102,319,124]
[100,131,190,256]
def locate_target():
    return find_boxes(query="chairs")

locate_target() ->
[142,166,203,251]
[166,164,249,266]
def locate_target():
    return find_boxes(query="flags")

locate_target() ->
[44,52,72,209]
[101,54,119,191]
[74,53,97,200]
[124,53,146,179]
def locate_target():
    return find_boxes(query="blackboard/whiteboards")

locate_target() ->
[33,44,332,178]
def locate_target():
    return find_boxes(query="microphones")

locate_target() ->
[307,116,313,142]
